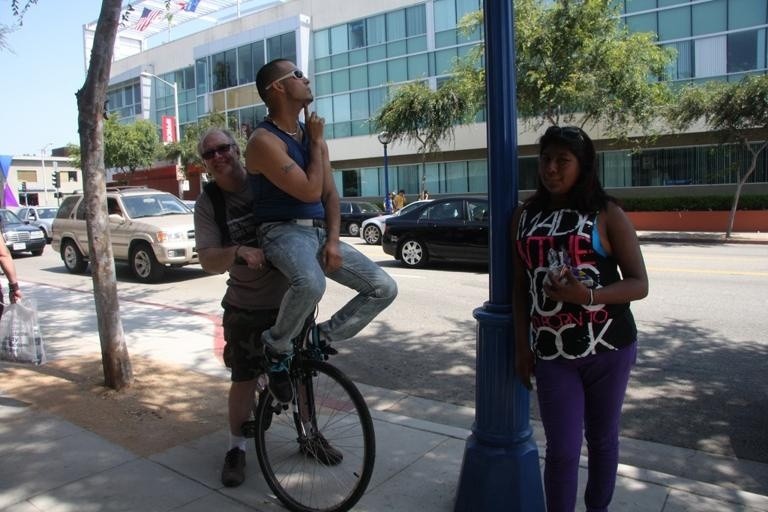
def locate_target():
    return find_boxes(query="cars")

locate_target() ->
[359,197,487,245]
[338,201,383,236]
[144,196,195,212]
[0,209,47,259]
[381,193,525,266]
[18,207,58,242]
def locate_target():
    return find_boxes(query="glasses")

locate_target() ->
[265,70,303,90]
[202,143,231,160]
[545,126,584,143]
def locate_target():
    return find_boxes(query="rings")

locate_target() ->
[258,264,262,268]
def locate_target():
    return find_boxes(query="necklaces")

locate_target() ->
[269,117,300,135]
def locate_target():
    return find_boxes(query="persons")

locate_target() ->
[418,190,428,201]
[517,125,648,512]
[384,190,407,212]
[0,230,23,322]
[193,129,344,488]
[243,58,398,403]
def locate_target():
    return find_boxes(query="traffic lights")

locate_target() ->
[51,171,60,188]
[22,182,26,192]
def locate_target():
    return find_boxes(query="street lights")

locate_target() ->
[377,131,393,214]
[41,142,57,208]
[140,70,185,200]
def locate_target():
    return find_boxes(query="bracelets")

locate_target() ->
[8,282,19,293]
[588,288,594,307]
[235,244,242,256]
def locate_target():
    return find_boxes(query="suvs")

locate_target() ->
[50,185,202,282]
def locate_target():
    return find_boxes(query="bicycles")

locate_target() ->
[237,309,377,511]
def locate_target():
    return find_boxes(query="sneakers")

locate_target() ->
[296,326,324,376]
[262,352,296,404]
[222,446,245,487]
[300,434,343,464]
